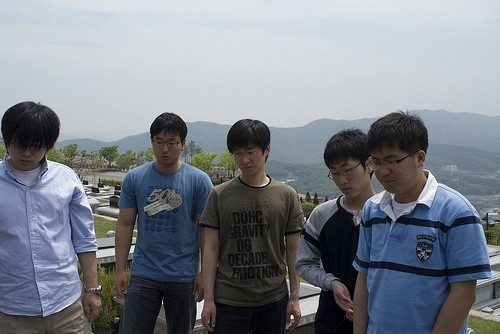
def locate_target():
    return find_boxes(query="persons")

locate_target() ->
[199,119,307,334]
[352,108,492,334]
[115,112,214,334]
[295,127,377,334]
[0,101,103,334]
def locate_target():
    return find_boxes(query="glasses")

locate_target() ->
[9,141,49,152]
[232,147,266,159]
[153,138,182,147]
[328,162,362,179]
[367,149,419,172]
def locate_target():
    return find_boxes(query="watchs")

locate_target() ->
[85,285,102,296]
